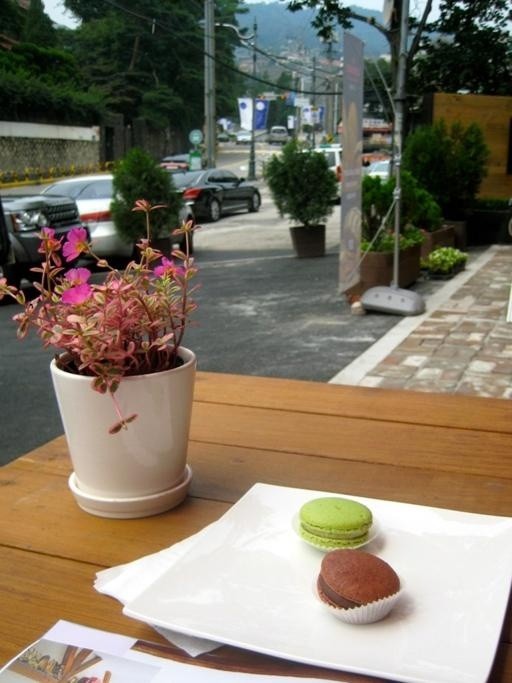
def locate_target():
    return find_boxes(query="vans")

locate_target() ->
[265,126,288,146]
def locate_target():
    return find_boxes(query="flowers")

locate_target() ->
[0,199,203,436]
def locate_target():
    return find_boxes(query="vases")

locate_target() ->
[49,344,197,519]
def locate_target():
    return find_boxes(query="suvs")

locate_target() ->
[0,187,92,304]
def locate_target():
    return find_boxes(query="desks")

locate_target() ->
[0,368,512,683]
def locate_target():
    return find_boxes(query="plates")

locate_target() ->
[122,481,511,683]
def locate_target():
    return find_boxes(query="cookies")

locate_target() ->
[316,549,401,610]
[298,497,373,548]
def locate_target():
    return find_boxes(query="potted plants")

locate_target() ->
[261,136,338,260]
[361,117,491,292]
[106,147,184,270]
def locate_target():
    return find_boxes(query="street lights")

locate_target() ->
[194,16,260,180]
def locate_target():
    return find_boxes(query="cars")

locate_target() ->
[38,151,260,263]
[300,137,399,207]
[216,133,230,143]
[234,128,253,144]
